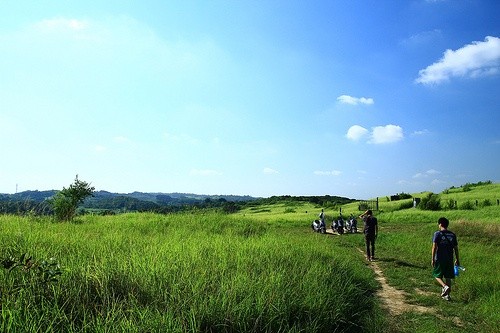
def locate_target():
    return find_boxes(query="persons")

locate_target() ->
[359,210,378,260]
[432,217,460,300]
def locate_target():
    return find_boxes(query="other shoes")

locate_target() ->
[371,256,375,259]
[366,257,371,261]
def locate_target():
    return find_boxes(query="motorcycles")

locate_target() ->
[344,213,358,234]
[310,209,327,234]
[331,216,344,236]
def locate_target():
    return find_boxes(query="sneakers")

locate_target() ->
[443,295,449,300]
[441,285,450,297]
